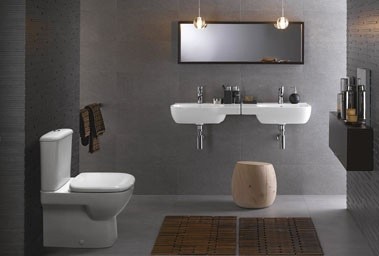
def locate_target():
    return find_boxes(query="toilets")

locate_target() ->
[39,128,136,248]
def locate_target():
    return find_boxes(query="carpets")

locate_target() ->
[150,216,237,256]
[239,217,324,256]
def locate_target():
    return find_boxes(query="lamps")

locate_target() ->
[194,0,208,30]
[274,0,289,30]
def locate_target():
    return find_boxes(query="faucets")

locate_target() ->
[196,86,203,102]
[278,86,285,102]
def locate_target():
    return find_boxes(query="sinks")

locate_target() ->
[170,102,227,125]
[257,102,312,124]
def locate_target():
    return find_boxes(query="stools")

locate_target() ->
[231,161,277,209]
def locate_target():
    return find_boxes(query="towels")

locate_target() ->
[80,108,91,146]
[84,103,105,154]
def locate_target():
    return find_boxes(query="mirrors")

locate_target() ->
[178,20,304,64]
